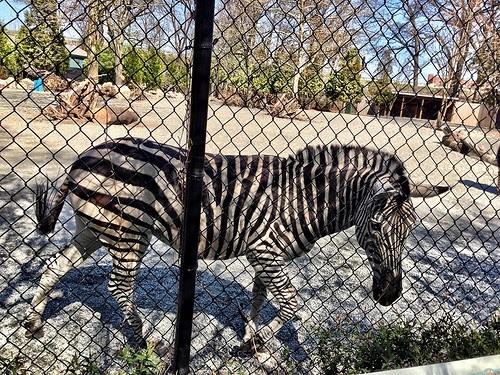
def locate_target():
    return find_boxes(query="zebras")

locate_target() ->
[24,136,453,360]
